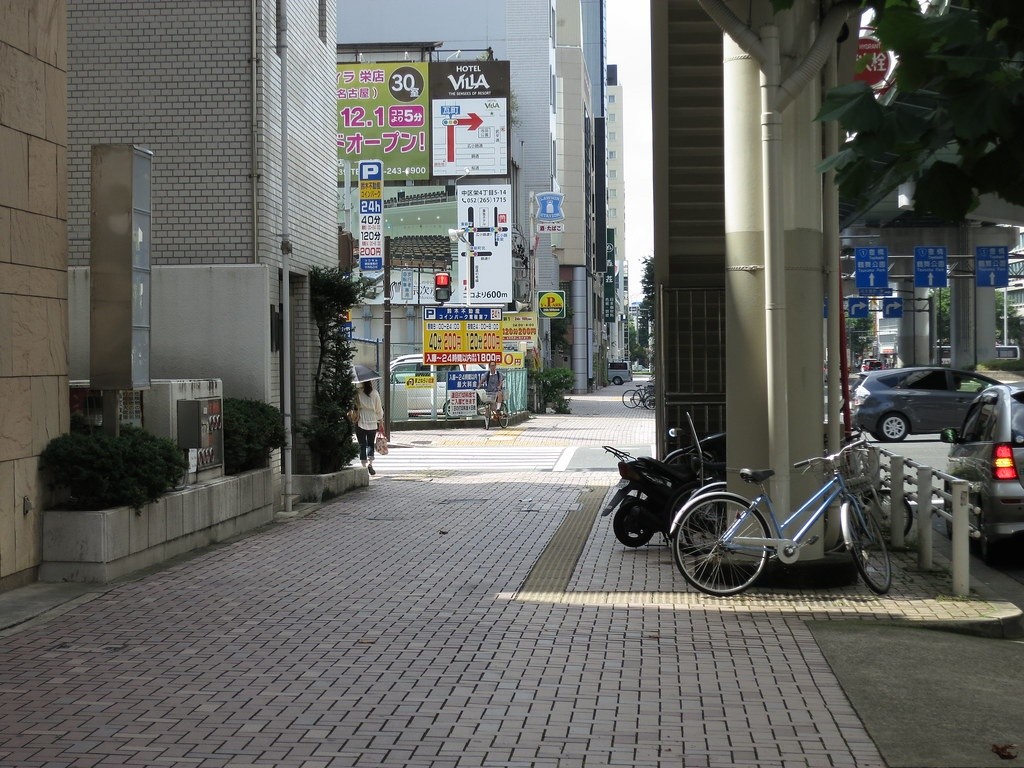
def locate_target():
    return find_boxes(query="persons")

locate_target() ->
[858,356,862,370]
[478,361,505,418]
[348,380,384,475]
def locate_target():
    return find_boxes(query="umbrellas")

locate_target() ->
[347,366,382,387]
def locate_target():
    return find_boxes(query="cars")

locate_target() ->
[839,367,1010,444]
[937,382,1024,565]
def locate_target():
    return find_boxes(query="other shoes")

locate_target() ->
[497,409,500,413]
[367,464,376,476]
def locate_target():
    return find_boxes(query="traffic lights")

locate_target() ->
[433,271,451,303]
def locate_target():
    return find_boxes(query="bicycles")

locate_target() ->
[670,442,893,598]
[826,428,913,540]
[669,458,729,559]
[621,384,656,411]
[473,388,509,430]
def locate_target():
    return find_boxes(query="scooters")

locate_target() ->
[597,427,728,549]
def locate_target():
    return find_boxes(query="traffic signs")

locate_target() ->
[914,246,947,288]
[855,247,890,289]
[976,247,1011,288]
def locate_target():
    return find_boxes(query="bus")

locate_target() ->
[895,344,1020,369]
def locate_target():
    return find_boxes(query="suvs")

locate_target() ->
[608,360,632,385]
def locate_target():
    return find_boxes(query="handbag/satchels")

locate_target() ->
[376,428,388,455]
[352,390,360,424]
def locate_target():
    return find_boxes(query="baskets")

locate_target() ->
[482,392,497,404]
[813,451,873,494]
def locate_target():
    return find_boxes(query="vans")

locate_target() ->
[390,354,492,417]
[859,359,882,372]
